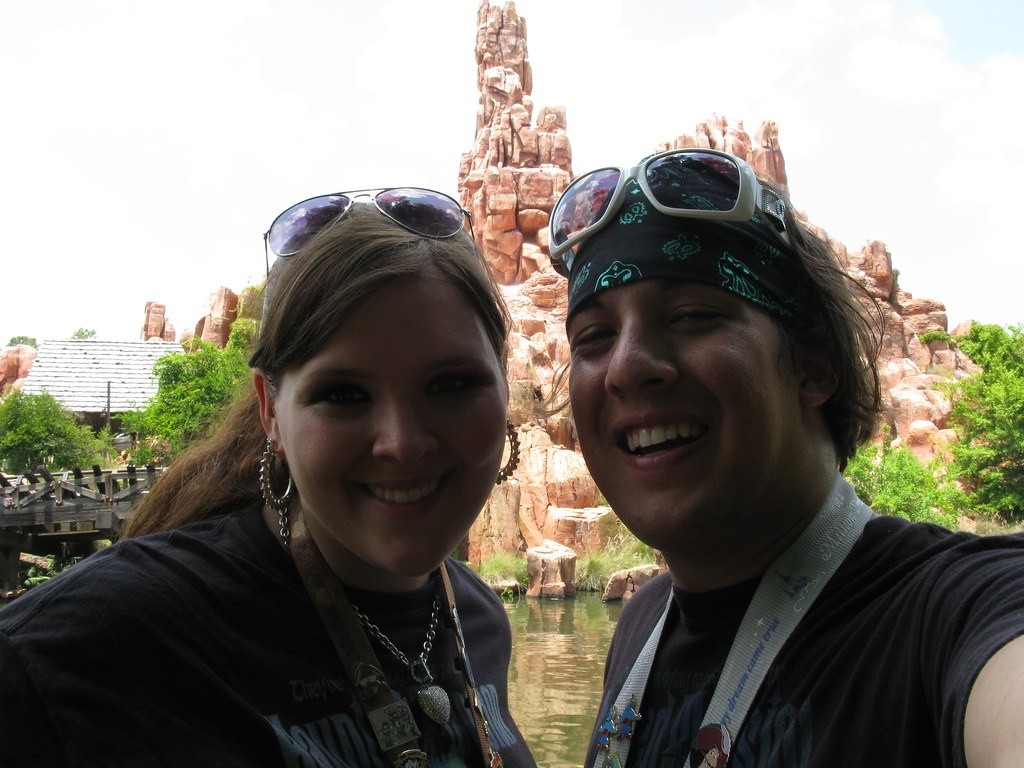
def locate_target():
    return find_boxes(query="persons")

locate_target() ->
[548,148,1024,768]
[0,186,539,768]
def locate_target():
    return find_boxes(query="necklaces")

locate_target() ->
[279,507,450,727]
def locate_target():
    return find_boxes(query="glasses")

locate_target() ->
[263,186,476,275]
[549,147,795,277]
